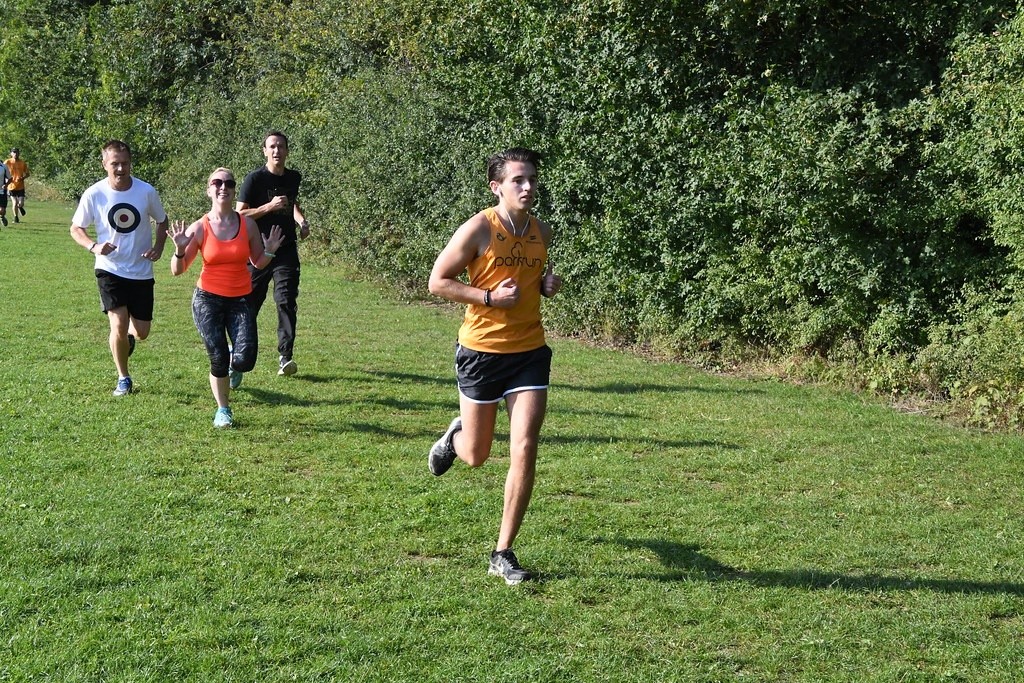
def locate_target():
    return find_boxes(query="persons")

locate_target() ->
[429,147,563,585]
[166,167,285,427]
[236,131,310,376]
[69,140,169,396]
[0,160,13,226]
[3,147,30,223]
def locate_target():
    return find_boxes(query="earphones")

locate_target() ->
[497,187,501,193]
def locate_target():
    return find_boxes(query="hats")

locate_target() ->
[11,148,19,152]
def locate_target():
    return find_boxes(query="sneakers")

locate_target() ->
[487,549,532,586]
[214,408,233,429]
[230,366,243,390]
[277,357,298,376]
[428,416,465,476]
[128,334,135,357]
[113,377,132,397]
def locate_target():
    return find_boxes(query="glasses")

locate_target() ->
[211,179,236,189]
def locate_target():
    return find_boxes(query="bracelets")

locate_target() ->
[174,252,185,258]
[88,241,97,253]
[263,250,277,258]
[484,289,492,307]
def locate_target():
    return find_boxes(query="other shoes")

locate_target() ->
[1,217,8,227]
[14,216,19,223]
[19,207,26,216]
